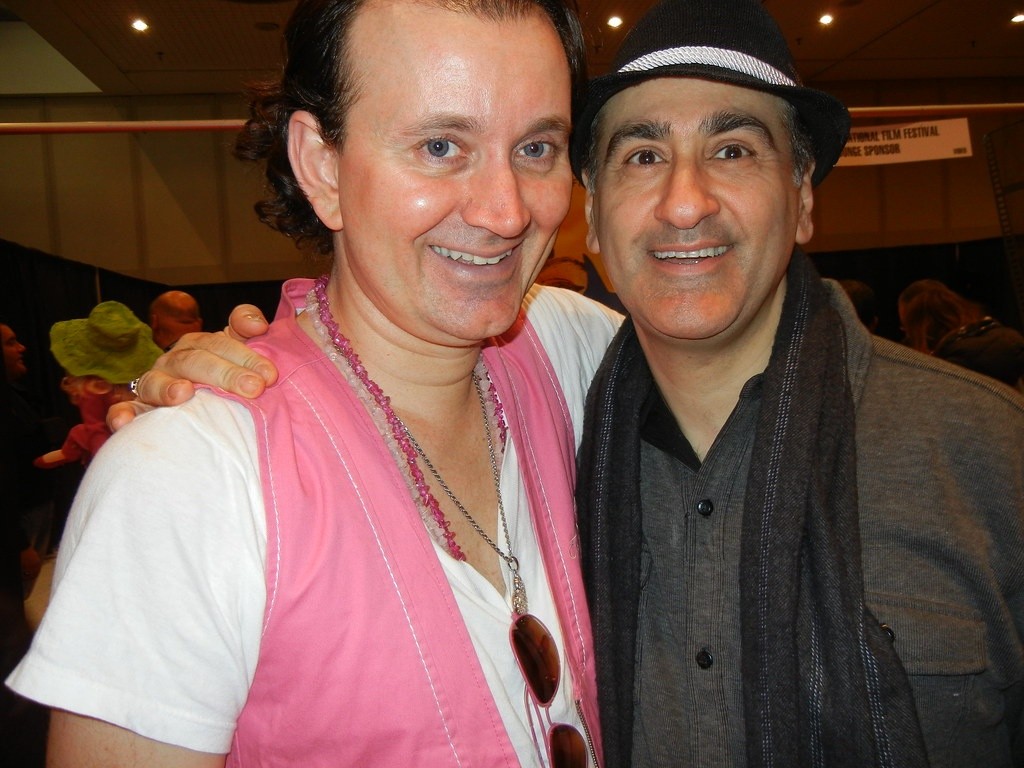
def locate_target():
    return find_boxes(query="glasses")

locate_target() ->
[509,612,589,768]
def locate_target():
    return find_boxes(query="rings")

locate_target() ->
[129,378,140,396]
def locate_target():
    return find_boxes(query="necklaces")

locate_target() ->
[306,274,528,616]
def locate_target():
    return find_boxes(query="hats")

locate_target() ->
[51,301,164,384]
[568,0,851,189]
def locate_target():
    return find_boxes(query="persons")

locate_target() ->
[104,0,1024,768]
[0,286,202,639]
[836,275,1024,394]
[0,0,629,768]
